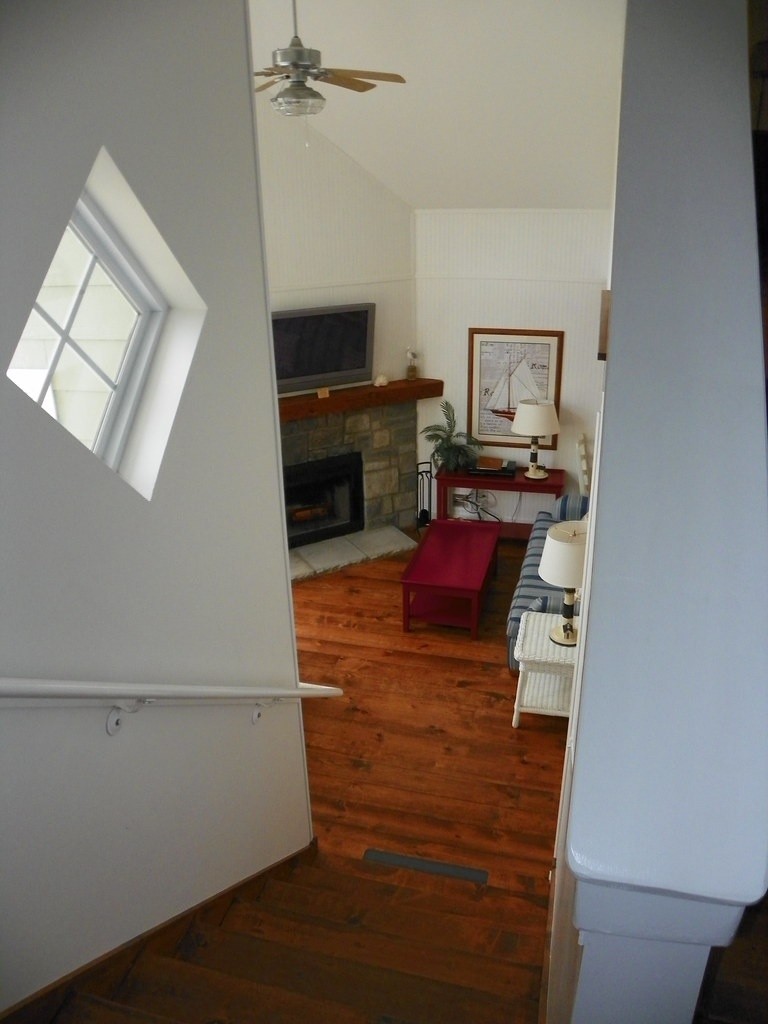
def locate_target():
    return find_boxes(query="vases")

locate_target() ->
[407,367,417,381]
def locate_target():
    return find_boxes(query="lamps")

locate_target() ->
[538,520,588,648]
[270,83,325,117]
[511,400,561,479]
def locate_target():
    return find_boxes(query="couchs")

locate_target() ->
[506,495,588,677]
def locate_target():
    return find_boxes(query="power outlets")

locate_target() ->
[480,492,488,508]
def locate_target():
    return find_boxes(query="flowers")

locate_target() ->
[407,350,417,367]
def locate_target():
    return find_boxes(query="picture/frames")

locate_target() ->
[467,328,564,453]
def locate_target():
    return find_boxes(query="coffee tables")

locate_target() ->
[398,519,502,641]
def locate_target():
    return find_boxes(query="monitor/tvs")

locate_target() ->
[273,303,376,398]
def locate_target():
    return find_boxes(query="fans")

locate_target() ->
[250,0,405,92]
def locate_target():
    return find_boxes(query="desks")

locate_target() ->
[512,612,579,729]
[434,462,566,519]
